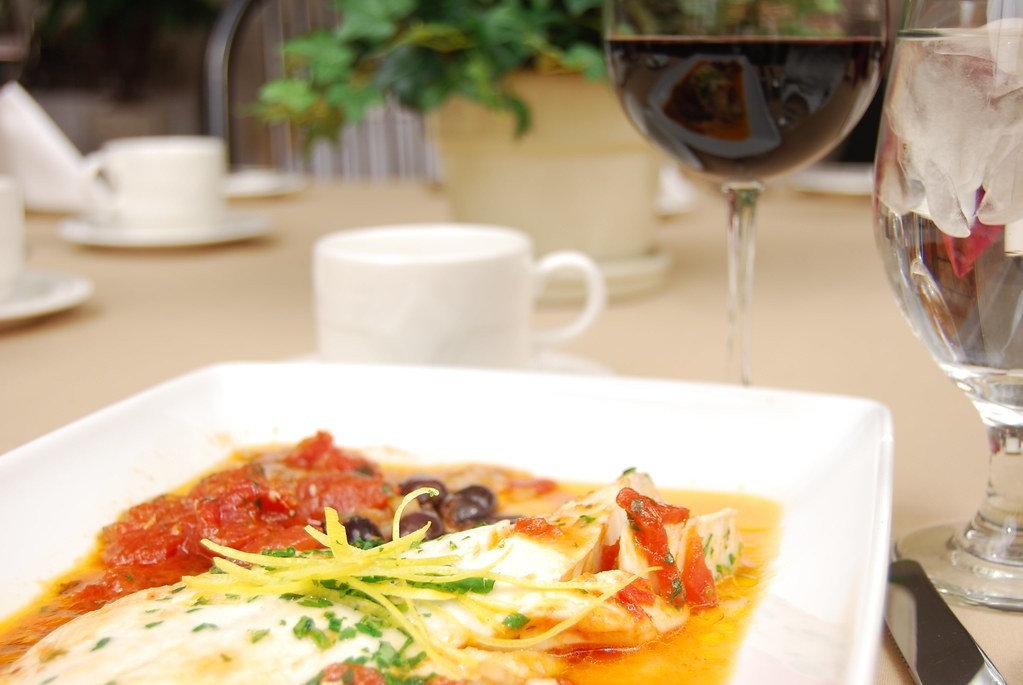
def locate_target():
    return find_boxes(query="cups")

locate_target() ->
[0,175,27,301]
[79,134,227,233]
[312,223,608,374]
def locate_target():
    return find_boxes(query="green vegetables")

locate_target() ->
[38,467,731,684]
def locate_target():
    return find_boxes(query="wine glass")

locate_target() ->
[604,1,884,387]
[869,24,1023,611]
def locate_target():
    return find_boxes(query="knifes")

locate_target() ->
[883,557,1009,685]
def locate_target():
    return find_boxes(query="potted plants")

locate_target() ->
[223,0,661,257]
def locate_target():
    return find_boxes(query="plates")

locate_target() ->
[1,361,895,685]
[60,206,273,248]
[1,271,98,328]
